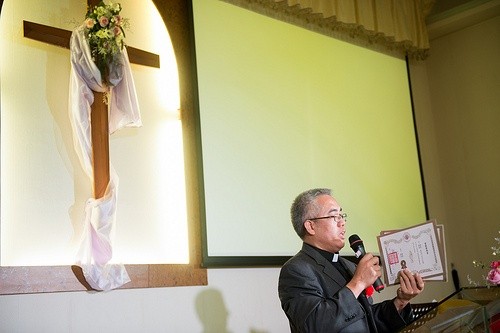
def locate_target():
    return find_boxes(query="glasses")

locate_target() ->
[303,213,347,226]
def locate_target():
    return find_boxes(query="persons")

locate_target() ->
[278,188,424,333]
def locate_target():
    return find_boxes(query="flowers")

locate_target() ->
[468,231,500,289]
[83,1,130,105]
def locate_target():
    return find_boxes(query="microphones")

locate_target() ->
[349,234,384,293]
